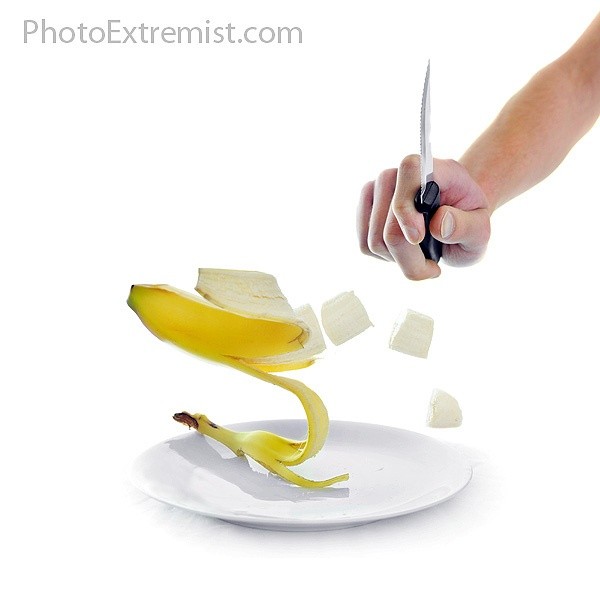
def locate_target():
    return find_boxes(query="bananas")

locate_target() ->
[126,268,349,495]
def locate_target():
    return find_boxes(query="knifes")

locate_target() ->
[412,59,443,264]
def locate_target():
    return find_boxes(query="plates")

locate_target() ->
[124,417,473,532]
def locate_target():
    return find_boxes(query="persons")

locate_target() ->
[356,12,600,281]
